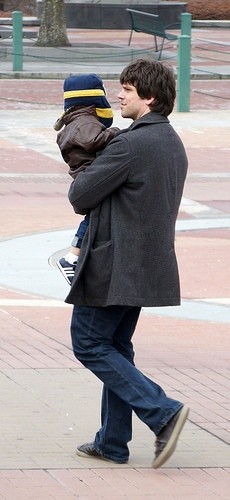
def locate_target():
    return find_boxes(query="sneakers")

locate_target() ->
[57,257,75,286]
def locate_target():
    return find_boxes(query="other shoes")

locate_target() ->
[77,442,130,463]
[151,407,190,467]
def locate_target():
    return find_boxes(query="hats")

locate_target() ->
[54,73,115,131]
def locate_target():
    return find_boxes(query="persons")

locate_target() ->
[64,60,188,470]
[53,72,120,286]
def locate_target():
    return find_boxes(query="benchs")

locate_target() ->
[126,8,179,61]
